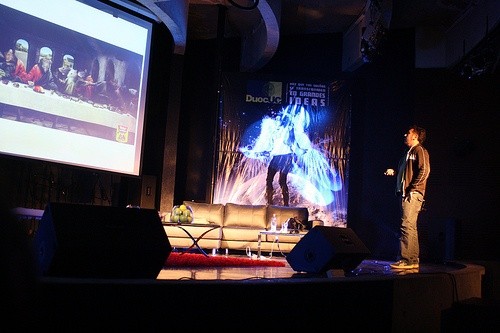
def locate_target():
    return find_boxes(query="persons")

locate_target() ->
[0,47,139,116]
[383,127,431,270]
[265,116,294,207]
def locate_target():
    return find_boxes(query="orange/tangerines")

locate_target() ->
[171,204,193,223]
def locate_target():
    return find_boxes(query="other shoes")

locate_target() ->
[390,259,420,269]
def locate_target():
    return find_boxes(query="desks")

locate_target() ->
[162,222,223,258]
[258,232,307,259]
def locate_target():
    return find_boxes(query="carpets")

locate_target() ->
[165,253,286,268]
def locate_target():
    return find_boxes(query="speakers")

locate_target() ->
[29,203,171,280]
[286,225,370,275]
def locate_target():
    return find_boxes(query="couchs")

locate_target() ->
[162,201,324,253]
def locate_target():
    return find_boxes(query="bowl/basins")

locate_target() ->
[170,205,194,224]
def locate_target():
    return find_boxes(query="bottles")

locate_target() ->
[271,214,277,232]
